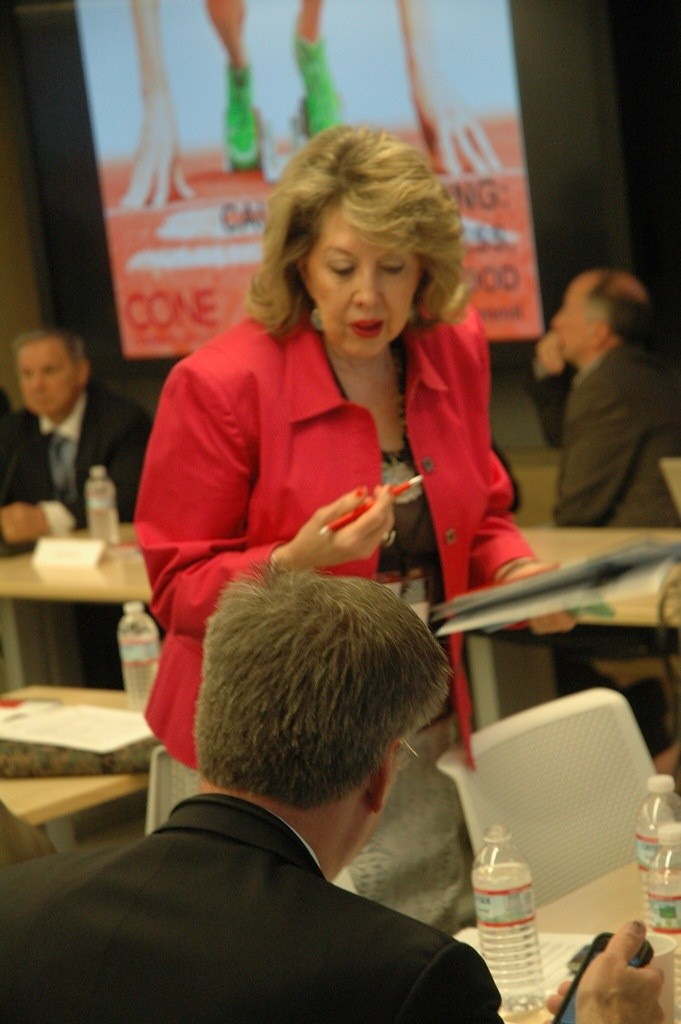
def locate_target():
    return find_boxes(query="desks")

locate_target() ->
[0,522,153,853]
[469,525,681,732]
[0,684,149,850]
[492,862,681,1024]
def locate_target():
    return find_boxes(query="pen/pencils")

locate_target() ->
[313,474,426,541]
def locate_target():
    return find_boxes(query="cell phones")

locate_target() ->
[552,932,654,1024]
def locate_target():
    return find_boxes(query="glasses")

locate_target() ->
[396,739,420,773]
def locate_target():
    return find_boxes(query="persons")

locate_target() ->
[1,329,155,692]
[529,266,681,773]
[133,126,574,938]
[119,0,500,206]
[0,557,663,1024]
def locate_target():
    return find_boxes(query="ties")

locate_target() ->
[47,435,71,508]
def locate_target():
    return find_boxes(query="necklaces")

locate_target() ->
[319,331,427,600]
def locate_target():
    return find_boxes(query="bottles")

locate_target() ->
[117,601,161,711]
[470,822,547,1016]
[634,772,681,881]
[641,821,681,1024]
[83,465,122,544]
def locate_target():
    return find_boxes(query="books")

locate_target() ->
[429,537,681,640]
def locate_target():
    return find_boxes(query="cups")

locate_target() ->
[644,932,678,1024]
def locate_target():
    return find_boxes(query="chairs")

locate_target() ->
[440,684,670,904]
